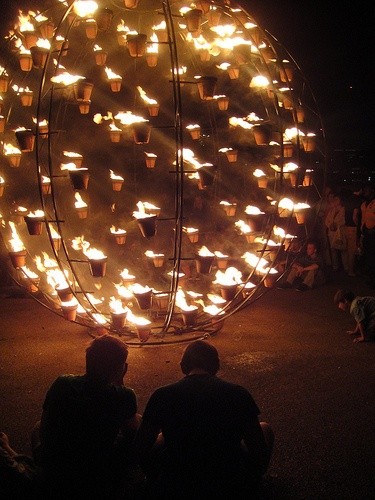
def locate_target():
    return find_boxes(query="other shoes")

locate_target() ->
[295,285,306,292]
[278,284,290,290]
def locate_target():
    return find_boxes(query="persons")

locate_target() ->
[333,288,375,344]
[279,240,329,293]
[136,341,276,500]
[32,335,143,500]
[323,183,375,278]
